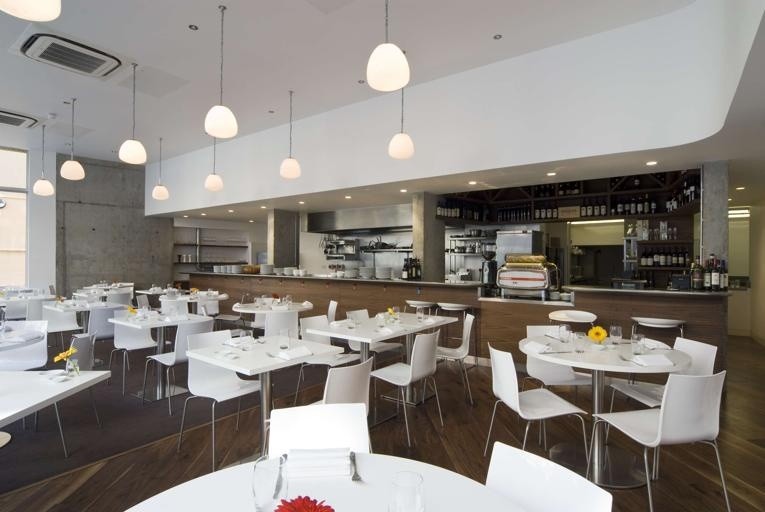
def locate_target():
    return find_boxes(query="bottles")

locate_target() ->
[102,289,108,307]
[690,254,729,292]
[580,174,697,267]
[401,258,422,281]
[437,182,579,222]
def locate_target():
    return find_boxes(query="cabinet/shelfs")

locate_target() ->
[436,169,701,290]
[173,241,248,265]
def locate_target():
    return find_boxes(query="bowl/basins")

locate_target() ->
[550,291,571,301]
[469,229,483,236]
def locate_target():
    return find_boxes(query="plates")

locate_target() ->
[214,265,243,274]
[331,267,392,279]
[260,264,307,277]
[40,369,67,384]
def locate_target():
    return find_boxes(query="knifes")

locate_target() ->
[272,453,287,500]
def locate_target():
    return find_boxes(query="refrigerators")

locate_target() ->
[496,230,543,270]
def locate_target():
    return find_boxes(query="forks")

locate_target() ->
[618,354,643,367]
[267,352,279,359]
[349,451,362,481]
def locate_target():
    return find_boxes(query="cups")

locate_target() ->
[71,296,77,307]
[190,288,214,301]
[19,288,38,298]
[0,320,4,343]
[240,327,288,352]
[384,471,426,512]
[137,305,151,321]
[347,306,423,332]
[254,294,293,310]
[181,254,192,262]
[150,283,173,292]
[251,454,289,512]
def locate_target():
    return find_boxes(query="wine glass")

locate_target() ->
[558,323,645,359]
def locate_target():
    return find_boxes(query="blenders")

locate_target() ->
[480,242,496,284]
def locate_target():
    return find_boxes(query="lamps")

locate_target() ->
[151,138,169,200]
[388,89,413,160]
[33,124,54,196]
[60,99,85,180]
[205,5,238,138]
[280,92,301,179]
[367,1,410,92]
[119,63,147,164]
[205,137,224,192]
